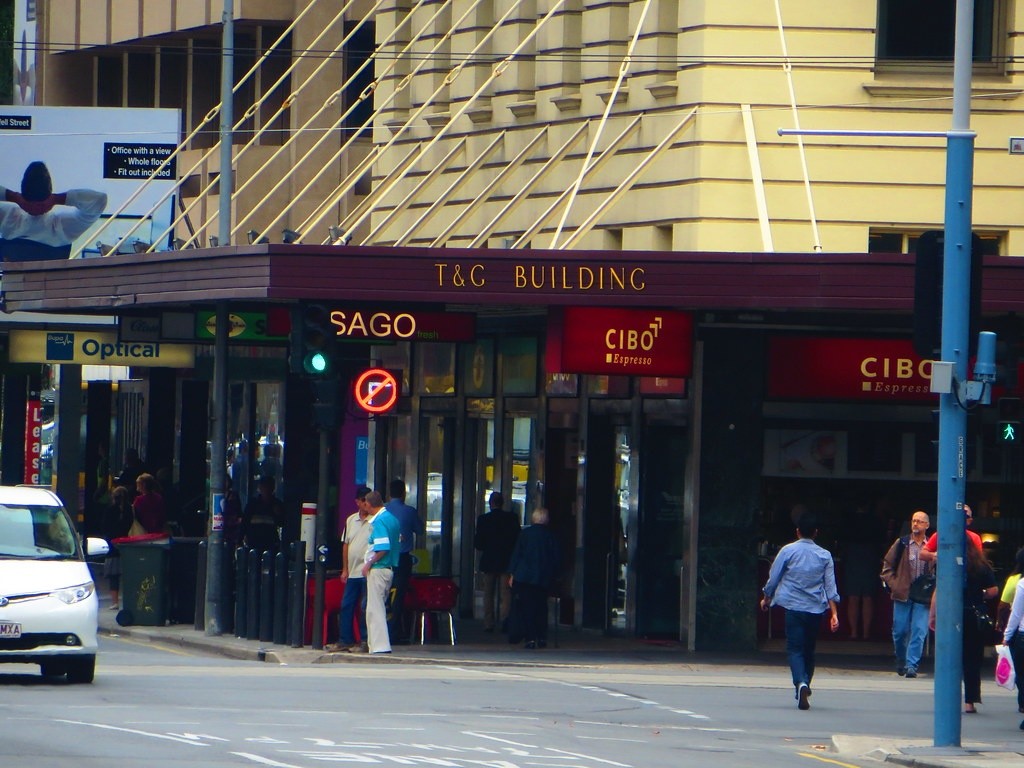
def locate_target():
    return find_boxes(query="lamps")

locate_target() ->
[96,241,119,256]
[209,235,218,247]
[247,230,269,244]
[328,226,352,245]
[173,238,194,250]
[281,229,300,243]
[132,241,155,253]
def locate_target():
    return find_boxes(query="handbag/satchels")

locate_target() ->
[995,643,1016,690]
[128,520,145,537]
[978,614,995,632]
[909,574,937,605]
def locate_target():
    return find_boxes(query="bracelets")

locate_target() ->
[369,558,376,564]
[983,589,986,599]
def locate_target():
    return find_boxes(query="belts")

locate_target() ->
[386,567,391,569]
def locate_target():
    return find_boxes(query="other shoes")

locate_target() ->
[797,682,812,709]
[527,640,545,649]
[484,628,493,633]
[110,604,119,609]
[907,668,917,678]
[1018,707,1024,714]
[1019,720,1024,730]
[327,641,357,652]
[897,659,907,676]
[965,703,977,713]
[502,619,508,633]
[360,642,368,653]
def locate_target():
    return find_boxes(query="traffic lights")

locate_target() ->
[299,298,336,378]
[997,396,1023,448]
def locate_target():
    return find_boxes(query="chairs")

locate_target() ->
[306,577,459,646]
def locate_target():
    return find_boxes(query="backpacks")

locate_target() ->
[883,536,910,594]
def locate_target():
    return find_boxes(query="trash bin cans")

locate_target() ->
[169,536,208,625]
[111,534,172,626]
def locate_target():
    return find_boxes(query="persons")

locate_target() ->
[961,533,999,713]
[879,511,930,678]
[383,480,424,646]
[996,549,1024,730]
[0,162,107,261]
[16,440,25,473]
[224,476,338,556]
[226,433,282,497]
[919,504,984,562]
[760,516,840,710]
[326,488,372,653]
[361,491,401,655]
[508,507,560,648]
[92,442,183,611]
[834,518,887,639]
[473,492,521,635]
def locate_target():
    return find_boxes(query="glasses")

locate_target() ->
[356,498,366,502]
[966,515,972,519]
[912,520,928,525]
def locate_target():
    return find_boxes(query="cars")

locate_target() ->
[0,481,112,686]
